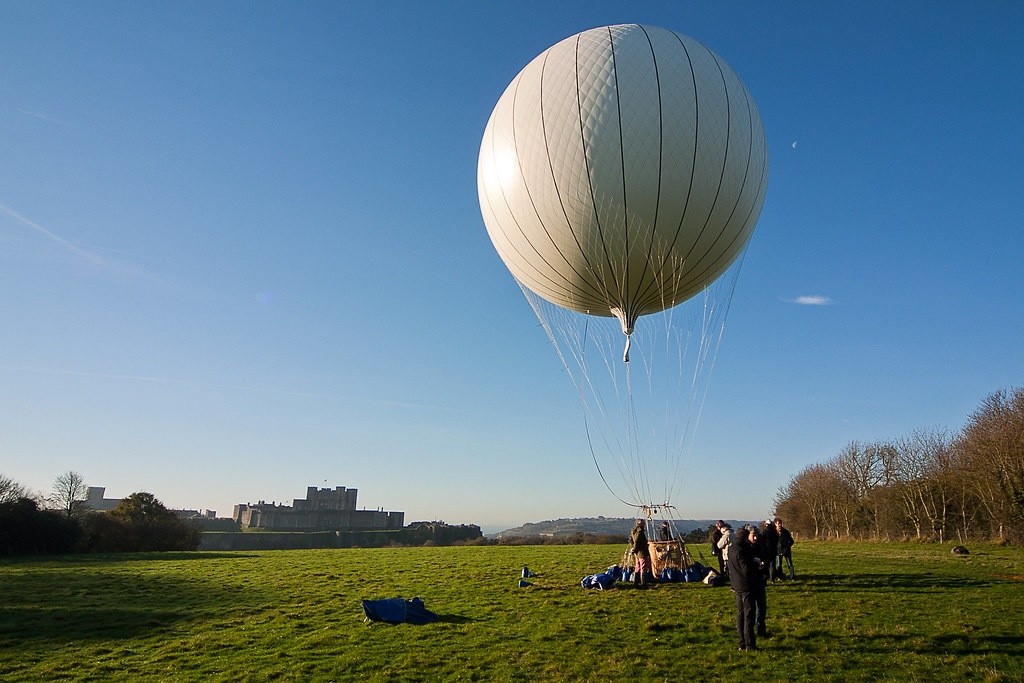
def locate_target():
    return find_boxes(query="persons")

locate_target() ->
[629,519,650,589]
[659,521,673,541]
[727,528,765,651]
[711,517,794,636]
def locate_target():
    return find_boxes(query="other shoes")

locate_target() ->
[746,644,759,652]
[778,576,782,580]
[770,578,774,584]
[790,577,795,582]
[757,632,773,639]
[738,645,746,651]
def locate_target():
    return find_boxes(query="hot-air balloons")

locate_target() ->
[476,22,767,579]
